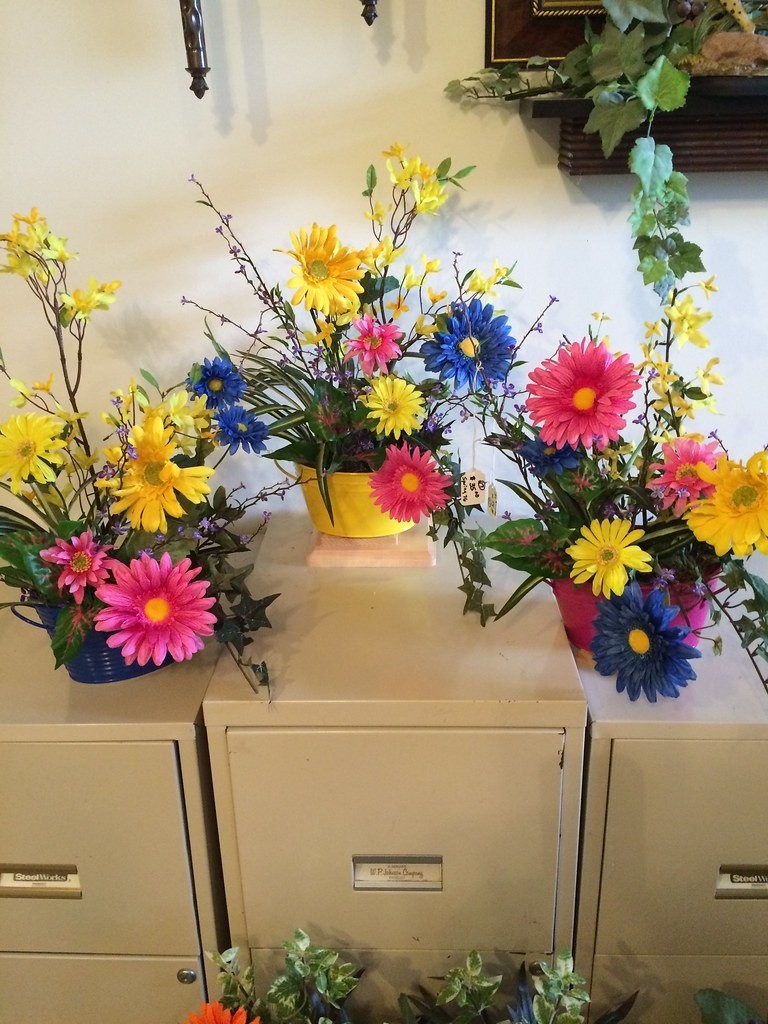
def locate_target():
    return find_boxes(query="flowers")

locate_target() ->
[182,927,642,1024]
[0,136,767,705]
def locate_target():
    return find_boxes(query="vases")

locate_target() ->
[23,592,173,684]
[274,456,416,539]
[555,564,725,658]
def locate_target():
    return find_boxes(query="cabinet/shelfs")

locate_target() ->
[570,592,768,1024]
[200,517,586,1024]
[0,533,263,1024]
